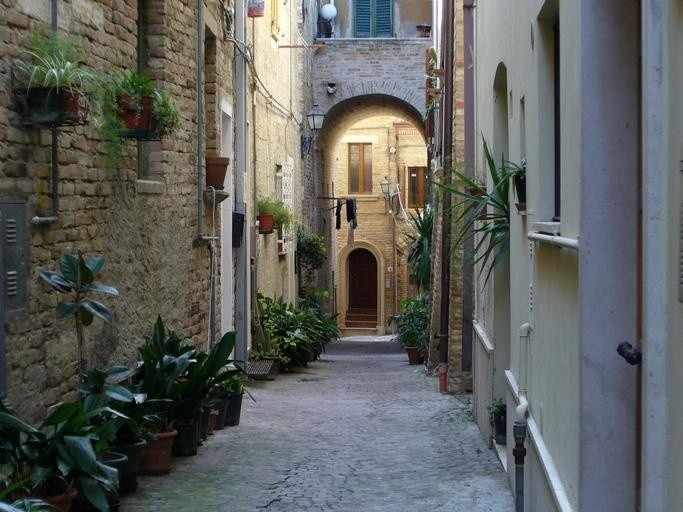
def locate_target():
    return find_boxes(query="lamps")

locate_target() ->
[317,3,338,37]
[301,101,325,159]
[380,175,393,200]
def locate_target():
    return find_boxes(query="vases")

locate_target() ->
[468,185,487,218]
[205,156,230,188]
[514,172,527,203]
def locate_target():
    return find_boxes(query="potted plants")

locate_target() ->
[485,396,505,445]
[255,289,284,376]
[0,325,246,509]
[388,294,430,365]
[258,197,291,234]
[8,23,183,179]
